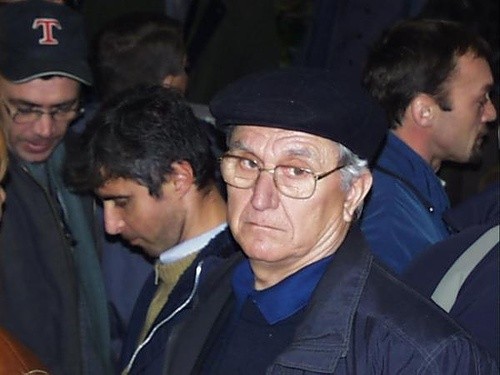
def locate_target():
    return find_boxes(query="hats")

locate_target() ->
[0,0,94,86]
[209,50,389,166]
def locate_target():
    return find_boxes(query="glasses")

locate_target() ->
[216,153,353,200]
[1,99,80,124]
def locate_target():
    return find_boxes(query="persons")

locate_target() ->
[348,16,500,279]
[0,99,53,375]
[160,64,499,375]
[397,184,500,375]
[0,1,115,375]
[69,12,189,140]
[56,81,241,375]
